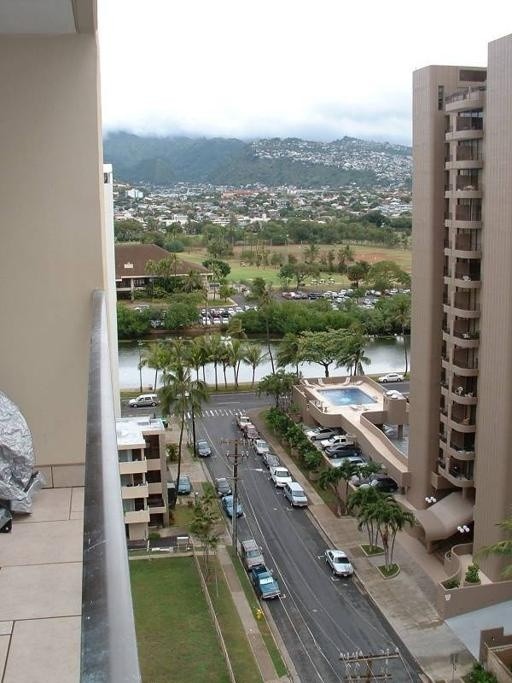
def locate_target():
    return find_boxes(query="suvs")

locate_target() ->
[244,424,260,440]
[128,393,161,407]
[320,434,354,451]
[367,477,398,492]
[238,537,265,569]
[343,455,369,467]
[282,482,308,507]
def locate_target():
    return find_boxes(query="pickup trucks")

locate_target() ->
[268,465,293,488]
[235,414,251,429]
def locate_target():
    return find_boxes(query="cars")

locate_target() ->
[281,286,411,308]
[222,496,244,517]
[173,390,190,399]
[133,304,256,328]
[250,564,280,600]
[378,372,405,382]
[263,452,280,468]
[305,426,340,440]
[214,477,233,496]
[323,442,362,458]
[252,438,269,455]
[174,475,192,493]
[324,548,355,577]
[195,438,213,456]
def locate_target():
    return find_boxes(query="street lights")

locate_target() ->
[456,524,470,542]
[424,495,437,506]
